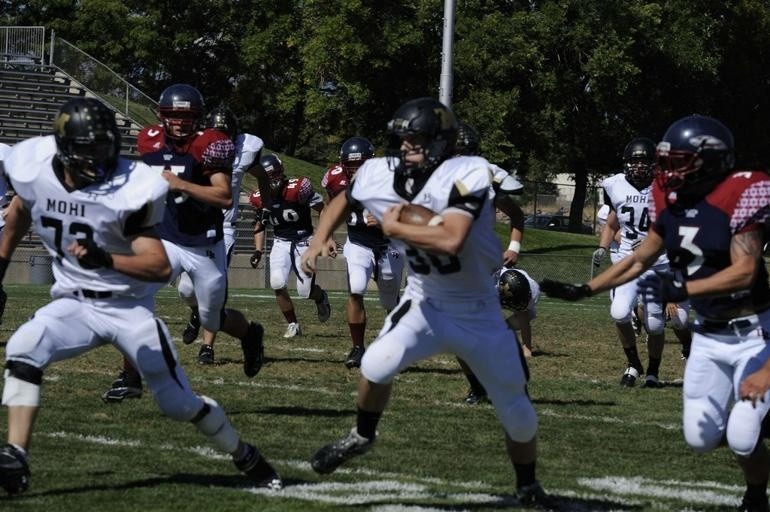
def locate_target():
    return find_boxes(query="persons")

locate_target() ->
[598,138,691,386]
[310,97,550,512]
[102,83,264,402]
[243,121,540,402]
[536,113,770,512]
[0,97,284,494]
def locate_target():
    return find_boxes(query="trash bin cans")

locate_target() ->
[29,255,54,285]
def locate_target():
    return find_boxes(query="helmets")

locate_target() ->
[158,82,207,141]
[501,271,532,310]
[55,97,122,184]
[385,99,456,178]
[455,124,481,155]
[207,110,238,142]
[621,138,656,185]
[259,153,284,190]
[658,117,737,216]
[340,137,375,179]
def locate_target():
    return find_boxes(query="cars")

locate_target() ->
[523,213,592,234]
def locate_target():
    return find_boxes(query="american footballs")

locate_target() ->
[391,204,444,225]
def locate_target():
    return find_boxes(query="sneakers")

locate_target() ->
[183,312,201,345]
[345,345,364,368]
[242,319,264,377]
[737,489,766,512]
[285,323,301,338]
[619,364,658,389]
[103,366,143,401]
[314,291,332,322]
[199,344,216,364]
[466,386,486,401]
[515,478,553,512]
[310,426,379,474]
[231,444,283,491]
[1,444,28,495]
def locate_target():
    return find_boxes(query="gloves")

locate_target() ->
[592,247,607,268]
[636,270,689,307]
[539,277,591,303]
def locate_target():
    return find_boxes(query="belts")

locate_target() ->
[74,289,113,299]
[704,318,752,333]
